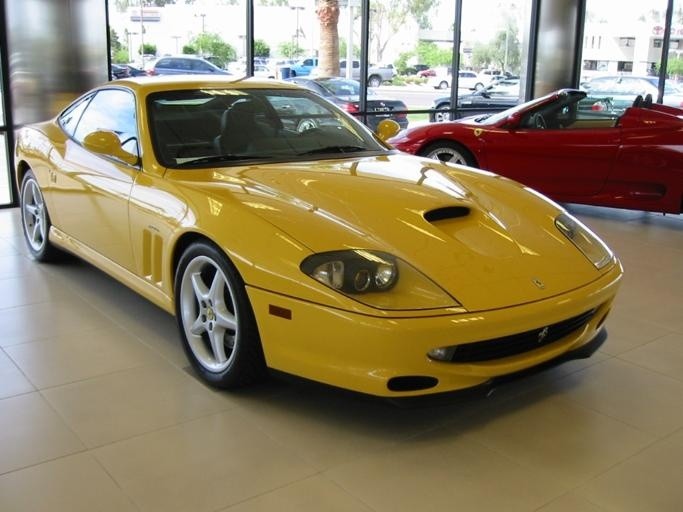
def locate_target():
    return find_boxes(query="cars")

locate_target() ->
[110,53,158,81]
[264,74,411,135]
[228,57,318,79]
[426,73,612,127]
[396,65,520,92]
[580,71,683,111]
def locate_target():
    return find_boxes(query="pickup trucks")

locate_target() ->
[339,58,394,88]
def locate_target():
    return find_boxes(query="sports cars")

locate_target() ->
[383,87,683,217]
[12,75,628,402]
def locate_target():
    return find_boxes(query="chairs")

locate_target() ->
[613,94,652,130]
[213,97,267,157]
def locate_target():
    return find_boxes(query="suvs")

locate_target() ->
[150,55,234,76]
